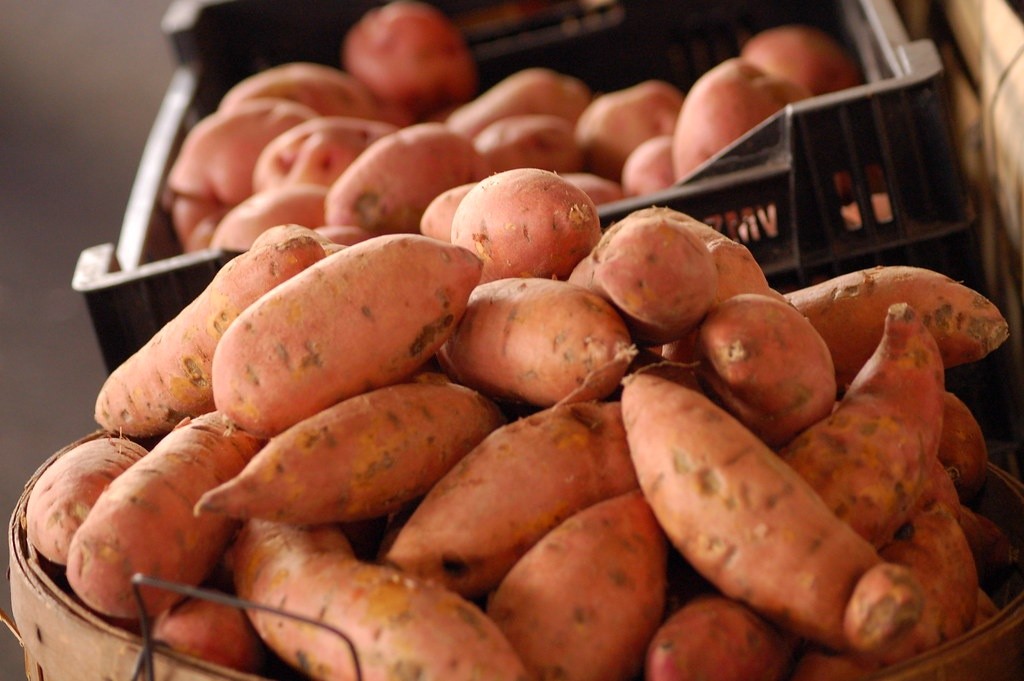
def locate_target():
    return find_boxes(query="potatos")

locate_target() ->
[30,168,1009,681]
[165,2,891,254]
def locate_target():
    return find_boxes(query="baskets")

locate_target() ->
[9,424,1021,681]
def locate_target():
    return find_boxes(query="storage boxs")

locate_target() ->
[71,0,1007,426]
